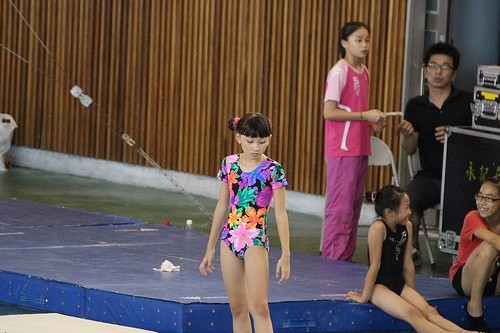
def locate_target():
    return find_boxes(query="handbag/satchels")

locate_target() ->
[0,113,19,173]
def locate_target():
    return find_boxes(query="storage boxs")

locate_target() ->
[438,65,500,264]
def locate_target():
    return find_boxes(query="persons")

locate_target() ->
[323,21,387,262]
[450,178,500,330]
[344,185,483,333]
[399,41,473,266]
[199,113,291,333]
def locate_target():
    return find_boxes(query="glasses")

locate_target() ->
[475,193,500,204]
[426,63,455,71]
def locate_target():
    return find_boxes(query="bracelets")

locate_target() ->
[360,112,363,120]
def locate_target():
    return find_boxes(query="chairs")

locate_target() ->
[318,135,437,270]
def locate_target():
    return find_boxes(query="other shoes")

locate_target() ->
[411,244,421,266]
[465,301,485,332]
